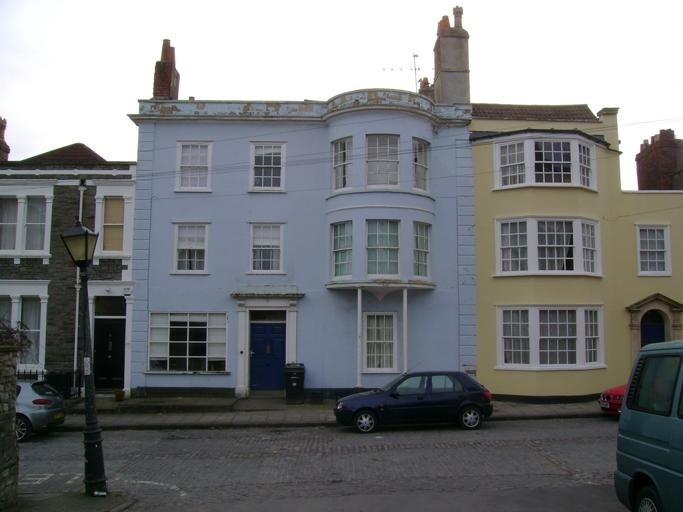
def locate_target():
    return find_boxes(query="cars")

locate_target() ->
[332,361,494,433]
[597,382,631,418]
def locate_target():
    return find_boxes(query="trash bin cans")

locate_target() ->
[284,362,305,404]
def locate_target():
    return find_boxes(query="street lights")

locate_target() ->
[57,213,111,499]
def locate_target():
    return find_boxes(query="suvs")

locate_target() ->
[14,379,66,442]
[609,338,683,510]
